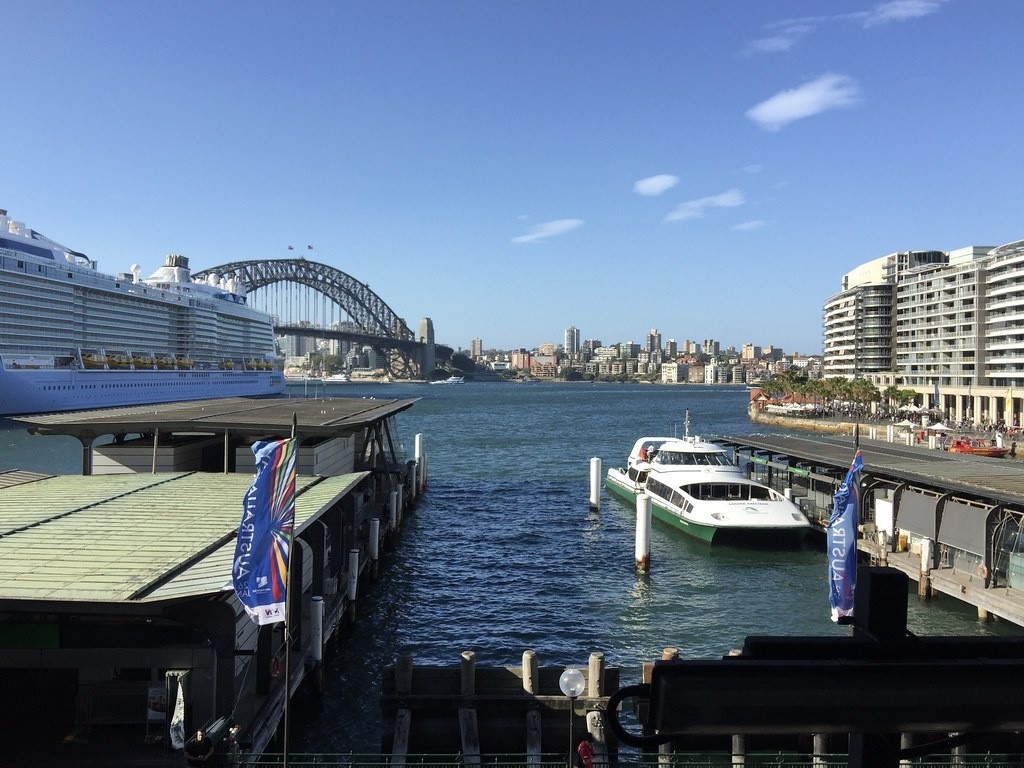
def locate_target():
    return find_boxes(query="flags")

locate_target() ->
[233,436,295,625]
[935,382,941,405]
[825,449,862,623]
[1006,387,1012,412]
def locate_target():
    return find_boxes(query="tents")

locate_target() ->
[899,404,931,413]
[894,419,917,431]
[927,423,953,434]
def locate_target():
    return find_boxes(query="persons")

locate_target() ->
[646,444,654,456]
[838,406,887,422]
[890,411,1024,436]
[577,733,595,768]
[184,728,215,768]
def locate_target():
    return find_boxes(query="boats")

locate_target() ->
[428,373,467,385]
[605,407,813,552]
[947,434,1011,457]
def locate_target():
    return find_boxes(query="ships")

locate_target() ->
[0,207,288,416]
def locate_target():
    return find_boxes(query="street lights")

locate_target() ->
[557,668,586,768]
